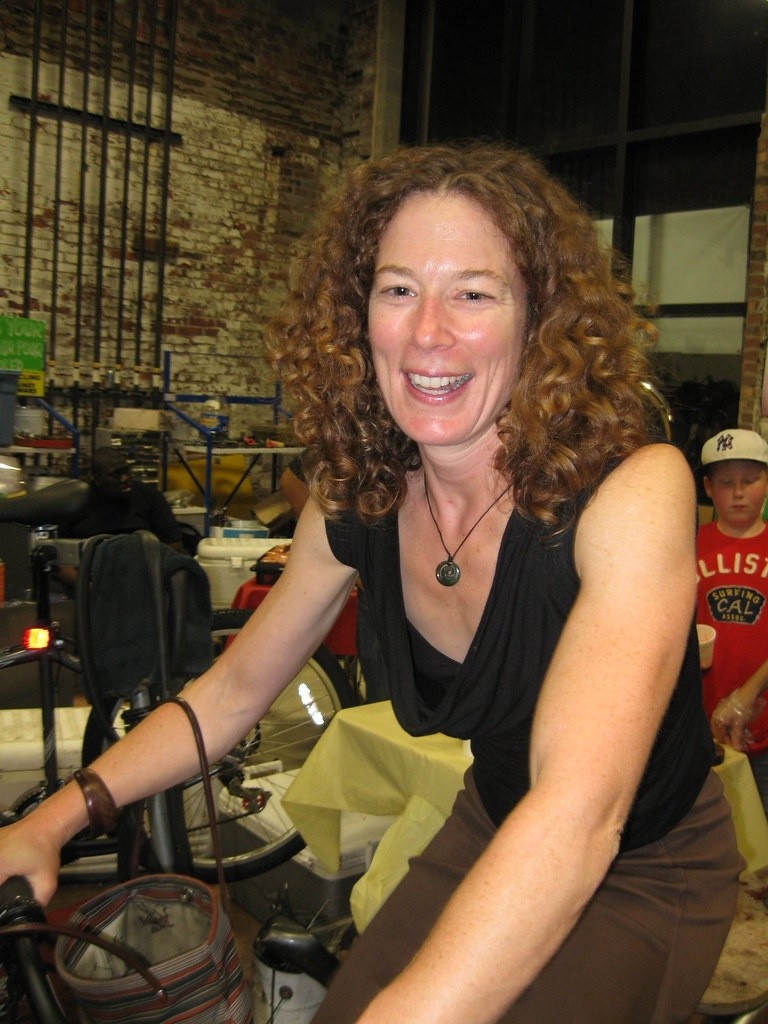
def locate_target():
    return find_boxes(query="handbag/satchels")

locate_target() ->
[0,696,256,1024]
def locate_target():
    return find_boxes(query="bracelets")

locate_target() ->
[74,767,119,834]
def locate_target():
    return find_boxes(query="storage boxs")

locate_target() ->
[218,760,401,932]
[170,490,294,611]
[113,407,174,431]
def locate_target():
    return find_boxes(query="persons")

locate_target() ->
[62,447,183,553]
[280,446,389,705]
[695,429,768,818]
[0,147,748,1024]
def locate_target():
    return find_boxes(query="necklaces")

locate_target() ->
[424,470,513,587]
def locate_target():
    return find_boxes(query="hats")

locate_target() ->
[701,429,768,467]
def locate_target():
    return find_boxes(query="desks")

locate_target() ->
[224,576,358,657]
[279,698,768,934]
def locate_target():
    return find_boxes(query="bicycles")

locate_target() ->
[0,475,768,1024]
